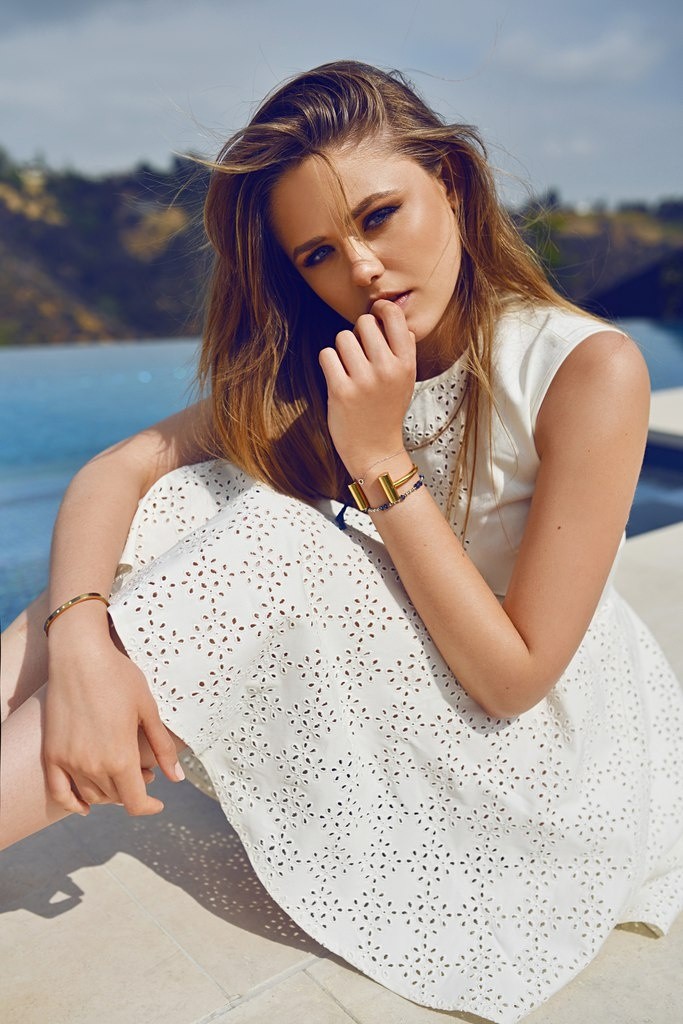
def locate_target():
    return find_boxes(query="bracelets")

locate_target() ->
[348,450,425,512]
[44,593,110,637]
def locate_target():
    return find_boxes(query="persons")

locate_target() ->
[0,61,683,1024]
